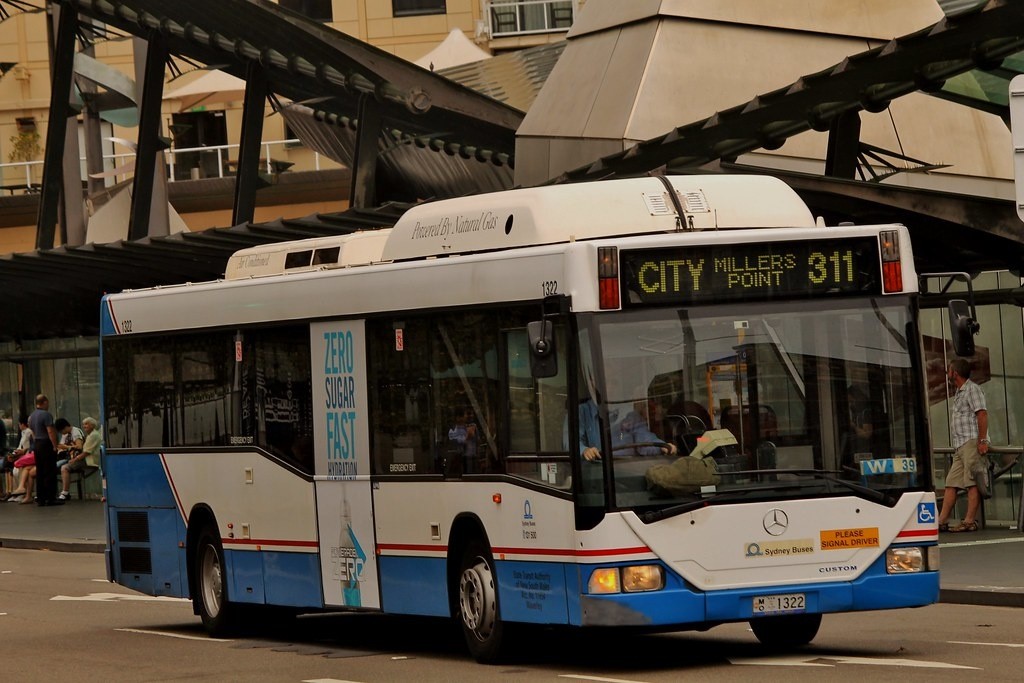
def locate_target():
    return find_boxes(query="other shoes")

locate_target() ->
[18,495,33,504]
[41,499,65,506]
[1,493,7,501]
[10,487,27,496]
[58,492,70,501]
[9,495,24,502]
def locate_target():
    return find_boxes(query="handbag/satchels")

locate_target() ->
[975,455,993,499]
[14,452,35,468]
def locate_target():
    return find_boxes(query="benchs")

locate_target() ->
[58,467,99,500]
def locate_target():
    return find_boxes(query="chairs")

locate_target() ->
[721,404,778,455]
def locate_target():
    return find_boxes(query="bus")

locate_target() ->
[98,173,982,665]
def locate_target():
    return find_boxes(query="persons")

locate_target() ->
[562,364,680,465]
[939,358,992,532]
[0,394,101,507]
[840,384,877,489]
[447,406,480,474]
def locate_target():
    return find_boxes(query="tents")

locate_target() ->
[412,27,494,72]
[160,68,268,113]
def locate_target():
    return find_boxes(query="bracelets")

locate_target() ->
[978,439,987,444]
[76,456,81,461]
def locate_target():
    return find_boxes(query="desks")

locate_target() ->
[226,159,296,175]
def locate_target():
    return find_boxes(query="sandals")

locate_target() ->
[939,521,948,531]
[948,521,978,532]
